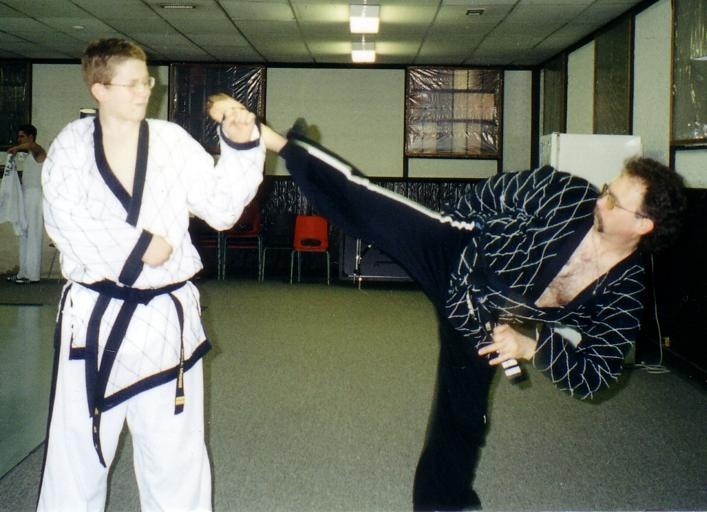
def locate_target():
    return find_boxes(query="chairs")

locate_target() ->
[287,214,335,285]
[187,202,276,284]
[348,229,411,288]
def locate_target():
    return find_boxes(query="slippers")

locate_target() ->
[7,274,19,281]
[15,277,40,284]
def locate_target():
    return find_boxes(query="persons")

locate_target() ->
[204,91,684,511]
[7,123,50,284]
[35,36,268,511]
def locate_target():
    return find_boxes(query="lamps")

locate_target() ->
[348,40,377,65]
[347,2,381,37]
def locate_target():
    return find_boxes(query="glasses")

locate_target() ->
[600,183,644,217]
[101,75,157,95]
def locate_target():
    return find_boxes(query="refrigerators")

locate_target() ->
[537,128,647,189]
